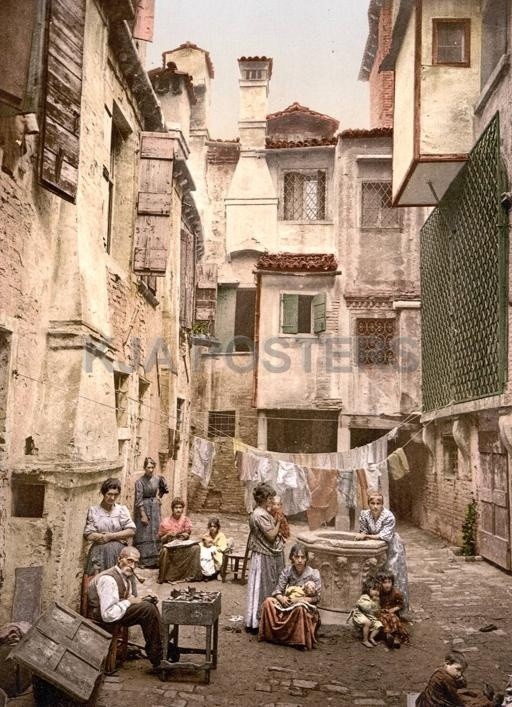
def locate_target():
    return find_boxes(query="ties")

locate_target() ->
[125,575,130,599]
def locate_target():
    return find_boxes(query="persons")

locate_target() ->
[415,650,504,707]
[156,497,201,584]
[200,535,217,581]
[83,478,137,575]
[135,457,169,568]
[376,572,405,648]
[257,543,322,650]
[354,492,409,617]
[266,581,318,608]
[246,483,285,629]
[352,578,382,648]
[268,495,289,540]
[88,546,175,673]
[200,518,227,580]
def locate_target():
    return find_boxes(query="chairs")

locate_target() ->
[80,573,128,674]
[221,531,252,585]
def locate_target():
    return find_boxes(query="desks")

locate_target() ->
[161,593,221,684]
